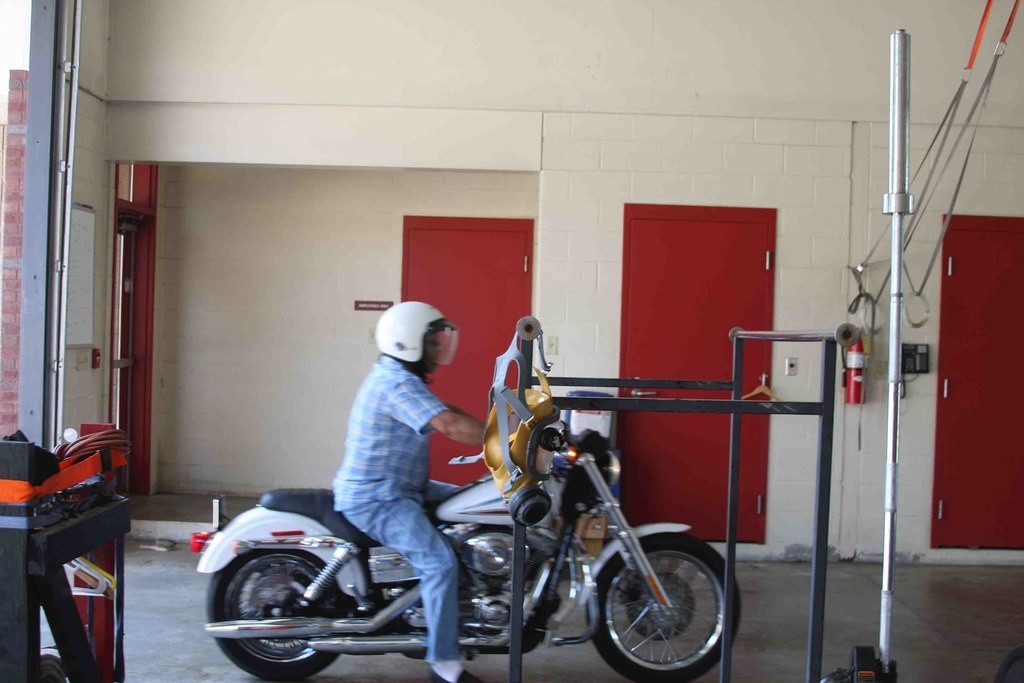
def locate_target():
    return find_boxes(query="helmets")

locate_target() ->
[375,301,459,374]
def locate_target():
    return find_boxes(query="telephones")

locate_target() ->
[901,343,930,373]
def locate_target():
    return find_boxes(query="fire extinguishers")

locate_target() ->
[841,336,868,405]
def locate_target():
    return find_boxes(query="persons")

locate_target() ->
[331,301,551,683]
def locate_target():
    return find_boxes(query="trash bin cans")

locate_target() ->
[551,447,623,503]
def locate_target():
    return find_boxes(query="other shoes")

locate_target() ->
[431,668,484,683]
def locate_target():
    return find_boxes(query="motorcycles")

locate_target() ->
[191,418,741,683]
